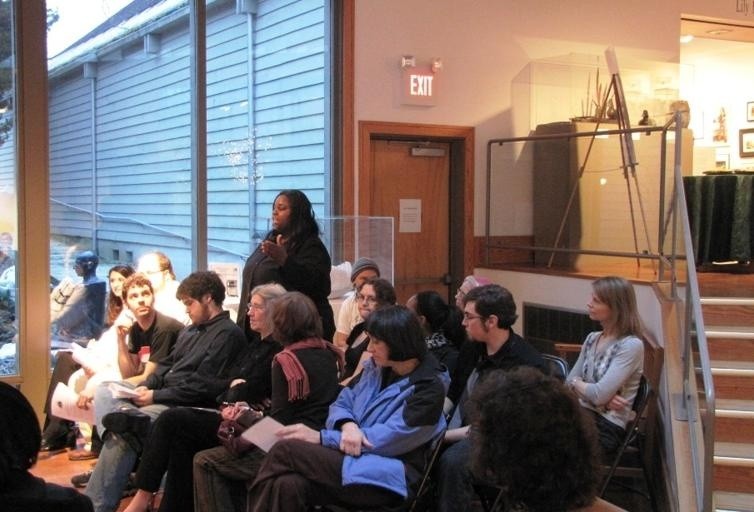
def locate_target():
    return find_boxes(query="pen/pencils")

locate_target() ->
[223,401,254,412]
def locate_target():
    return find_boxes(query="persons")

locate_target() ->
[238,188,336,345]
[0,231,647,512]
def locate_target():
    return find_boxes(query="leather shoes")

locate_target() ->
[40,428,77,452]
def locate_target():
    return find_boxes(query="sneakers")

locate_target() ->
[68,441,98,461]
[71,471,94,488]
[122,472,139,498]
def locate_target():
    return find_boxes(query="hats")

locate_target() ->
[350,256,381,283]
[464,275,493,288]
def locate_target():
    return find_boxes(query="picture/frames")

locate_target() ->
[739,127,754,157]
[746,101,754,121]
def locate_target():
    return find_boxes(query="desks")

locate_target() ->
[680,174,754,266]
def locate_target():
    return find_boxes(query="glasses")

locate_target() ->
[355,295,377,305]
[463,312,488,321]
[246,302,265,311]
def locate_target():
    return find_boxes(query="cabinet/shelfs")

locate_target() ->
[534,122,695,273]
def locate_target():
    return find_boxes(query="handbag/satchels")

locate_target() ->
[215,419,258,460]
